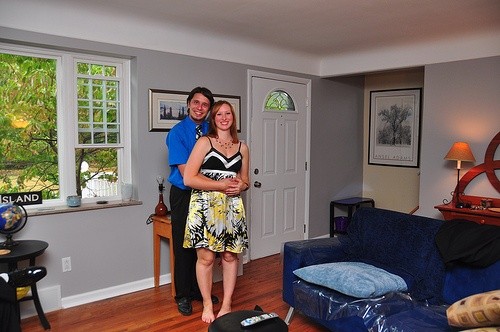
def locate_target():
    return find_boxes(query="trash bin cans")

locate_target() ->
[335,216,350,232]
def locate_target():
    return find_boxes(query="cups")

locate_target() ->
[67,195,81,207]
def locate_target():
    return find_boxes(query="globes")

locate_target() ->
[0,200,28,248]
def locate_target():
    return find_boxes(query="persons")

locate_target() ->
[165,87,218,315]
[182,100,250,324]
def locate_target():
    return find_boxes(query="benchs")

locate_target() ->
[281,206,500,332]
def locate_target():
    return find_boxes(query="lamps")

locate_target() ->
[443,141,476,208]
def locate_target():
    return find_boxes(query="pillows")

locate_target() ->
[445,289,500,328]
[292,261,408,299]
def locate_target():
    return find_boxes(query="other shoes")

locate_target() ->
[176,296,192,315]
[190,290,219,304]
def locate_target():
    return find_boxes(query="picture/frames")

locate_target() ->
[367,87,420,169]
[148,88,241,134]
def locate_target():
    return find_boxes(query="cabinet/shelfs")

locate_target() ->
[434,131,500,226]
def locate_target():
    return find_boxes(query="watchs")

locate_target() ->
[243,181,248,191]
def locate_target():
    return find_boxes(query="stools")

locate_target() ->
[207,310,289,332]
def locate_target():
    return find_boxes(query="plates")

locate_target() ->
[97,201,108,204]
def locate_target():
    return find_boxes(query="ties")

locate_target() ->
[195,125,202,142]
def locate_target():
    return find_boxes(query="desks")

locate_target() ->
[0,239,50,329]
[150,215,175,296]
[329,196,377,237]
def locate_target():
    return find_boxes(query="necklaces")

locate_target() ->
[215,133,233,150]
